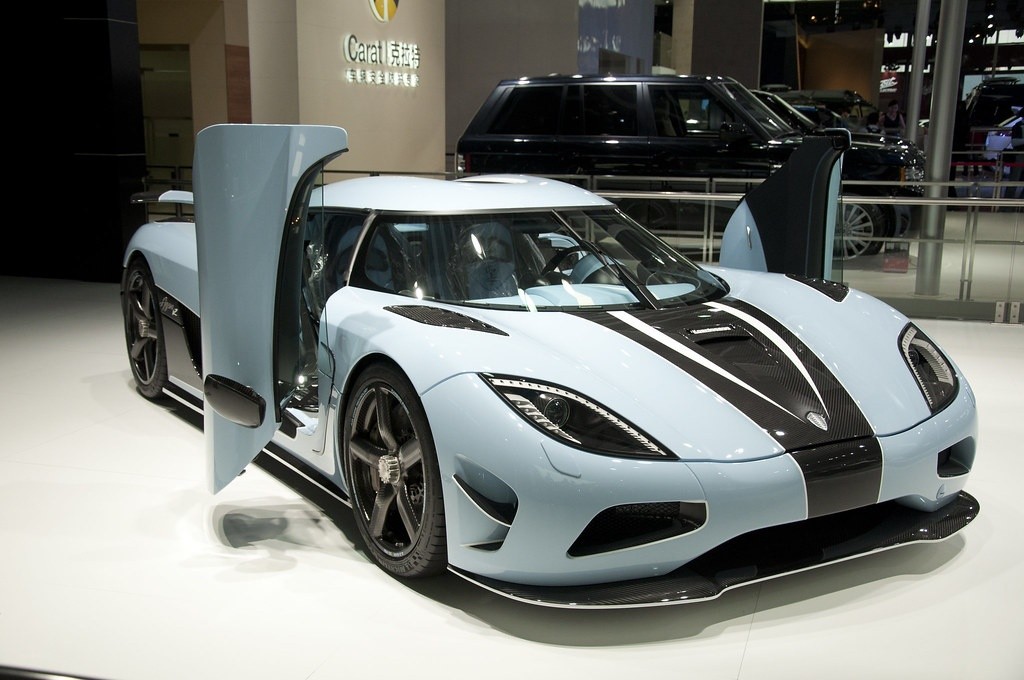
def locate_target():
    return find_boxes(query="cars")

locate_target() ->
[747,82,906,139]
[985,107,1023,179]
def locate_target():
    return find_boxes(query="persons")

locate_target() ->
[954,100,972,163]
[878,99,906,144]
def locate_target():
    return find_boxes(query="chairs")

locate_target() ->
[670,99,688,137]
[446,219,518,300]
[323,216,407,294]
[662,99,677,137]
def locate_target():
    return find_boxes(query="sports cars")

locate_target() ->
[118,122,983,613]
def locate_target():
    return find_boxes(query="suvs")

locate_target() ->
[455,71,931,271]
[964,75,1023,137]
